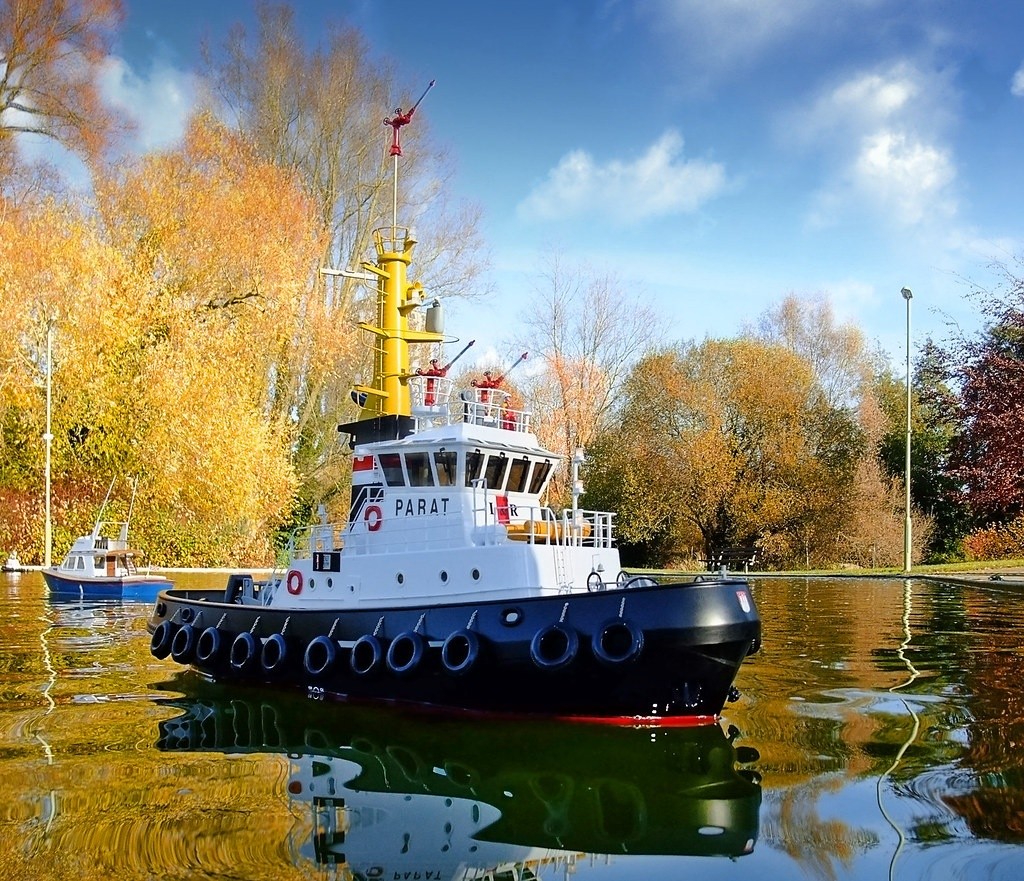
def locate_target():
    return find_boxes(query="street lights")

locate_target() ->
[43,312,57,567]
[900,287,911,573]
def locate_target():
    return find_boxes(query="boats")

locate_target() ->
[145,671,764,881]
[145,77,764,685]
[40,475,177,601]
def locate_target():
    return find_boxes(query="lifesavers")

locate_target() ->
[366,866,382,878]
[303,636,335,674]
[592,617,644,666]
[261,633,285,668]
[350,635,381,675]
[287,570,302,595]
[442,629,478,675]
[171,624,193,657]
[196,626,220,660]
[530,623,579,671]
[150,620,171,660]
[364,506,382,531]
[230,631,256,667]
[386,631,423,674]
